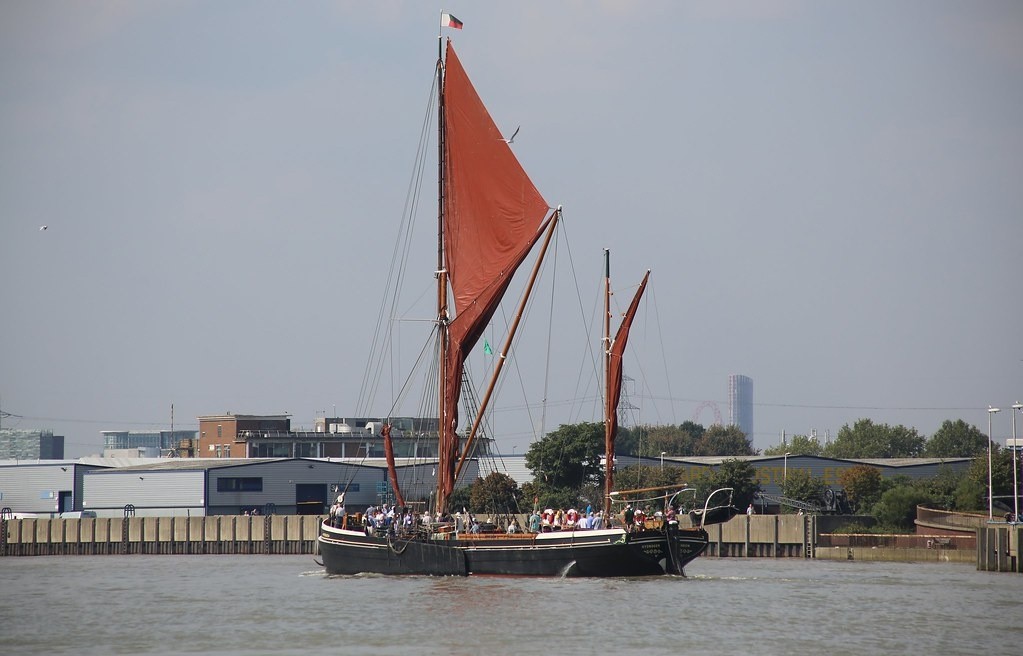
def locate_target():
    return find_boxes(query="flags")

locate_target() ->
[485,340,492,355]
[441,14,463,29]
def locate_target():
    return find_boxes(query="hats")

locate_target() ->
[590,512,594,515]
[670,506,673,509]
[596,513,600,516]
[537,511,541,515]
[472,518,477,522]
[626,505,631,508]
[487,518,492,523]
[454,512,461,516]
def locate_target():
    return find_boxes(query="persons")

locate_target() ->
[508,501,676,534]
[747,504,756,515]
[334,503,479,537]
[678,505,684,514]
[797,509,803,515]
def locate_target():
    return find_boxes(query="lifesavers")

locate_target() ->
[553,509,565,526]
[634,510,644,526]
[567,509,579,524]
[542,509,554,524]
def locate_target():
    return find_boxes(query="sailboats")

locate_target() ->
[318,7,708,578]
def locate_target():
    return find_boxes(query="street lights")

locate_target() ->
[1011,403,1023,521]
[783,452,790,498]
[988,407,1000,521]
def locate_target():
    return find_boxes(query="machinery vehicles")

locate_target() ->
[757,488,852,516]
